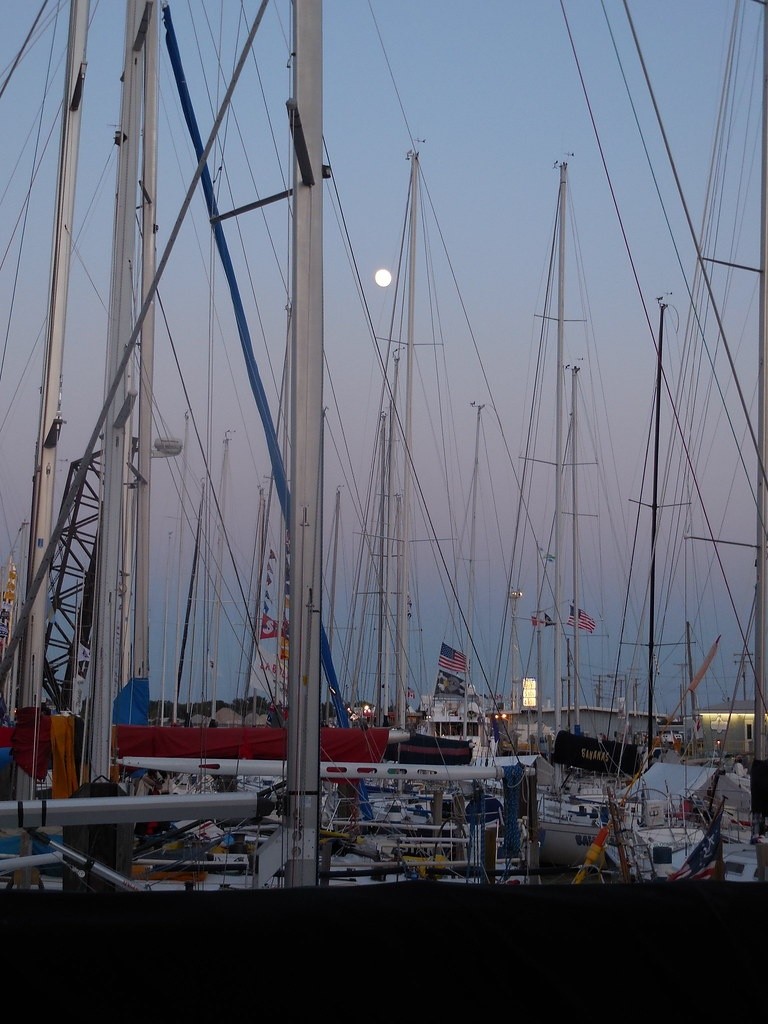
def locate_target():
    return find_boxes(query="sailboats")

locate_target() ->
[2,0,767,889]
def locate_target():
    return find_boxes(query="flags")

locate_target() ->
[264,549,277,614]
[250,646,288,698]
[567,605,596,634]
[437,642,471,673]
[530,609,554,627]
[408,690,415,698]
[260,613,279,639]
[433,669,470,698]
[667,811,723,880]
